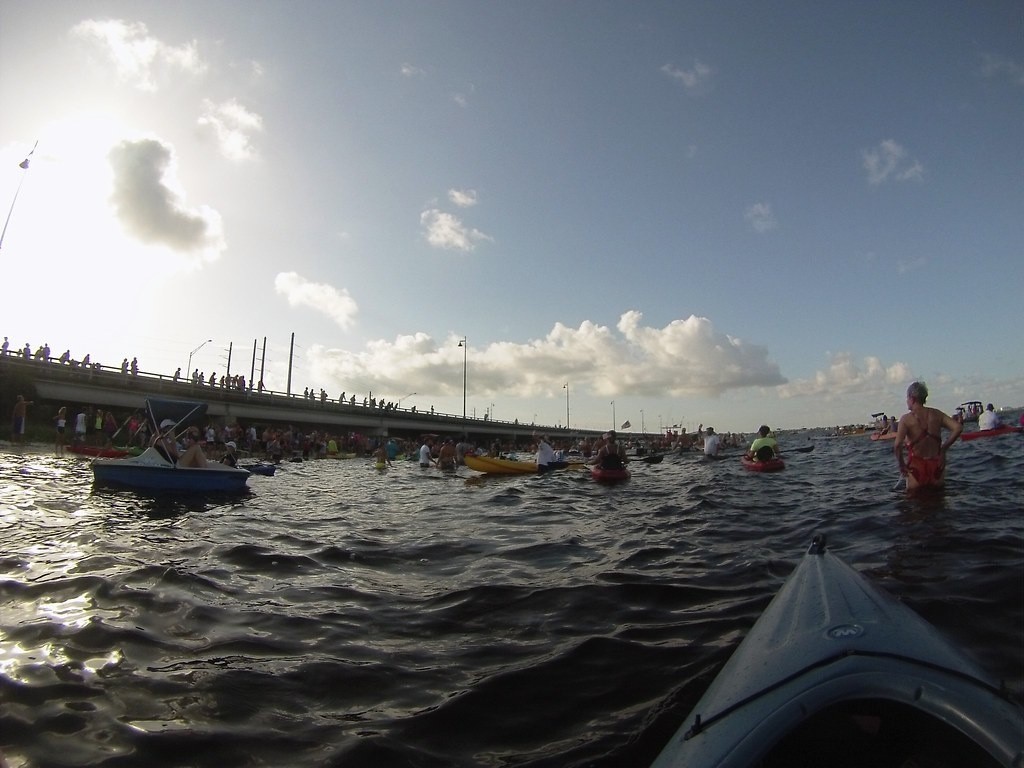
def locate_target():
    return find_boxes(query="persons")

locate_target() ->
[338,391,398,411]
[150,419,209,468]
[74,407,119,449]
[335,429,402,469]
[630,423,782,463]
[398,434,520,471]
[121,356,139,375]
[202,420,257,466]
[53,406,66,455]
[892,379,964,489]
[515,418,519,424]
[10,395,35,444]
[484,414,489,422]
[430,405,434,415]
[125,412,149,451]
[0,336,90,368]
[261,424,338,464]
[519,429,634,485]
[881,413,898,434]
[304,387,328,402]
[173,367,266,393]
[961,401,1024,431]
[412,406,416,412]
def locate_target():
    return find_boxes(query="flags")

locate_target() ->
[621,421,631,429]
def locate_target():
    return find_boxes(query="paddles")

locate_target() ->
[703,446,816,461]
[547,455,664,470]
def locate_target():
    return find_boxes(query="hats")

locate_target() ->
[225,441,237,452]
[160,419,177,429]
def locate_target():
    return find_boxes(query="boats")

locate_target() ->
[591,453,632,481]
[235,460,279,479]
[959,423,1024,440]
[741,445,785,472]
[955,401,984,423]
[89,448,253,494]
[871,431,898,440]
[65,443,130,456]
[464,450,586,473]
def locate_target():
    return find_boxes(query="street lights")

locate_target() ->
[610,400,616,432]
[490,402,496,423]
[563,381,570,430]
[640,409,644,436]
[398,392,418,409]
[458,336,467,418]
[186,339,214,382]
[659,414,663,436]
[533,413,538,424]
